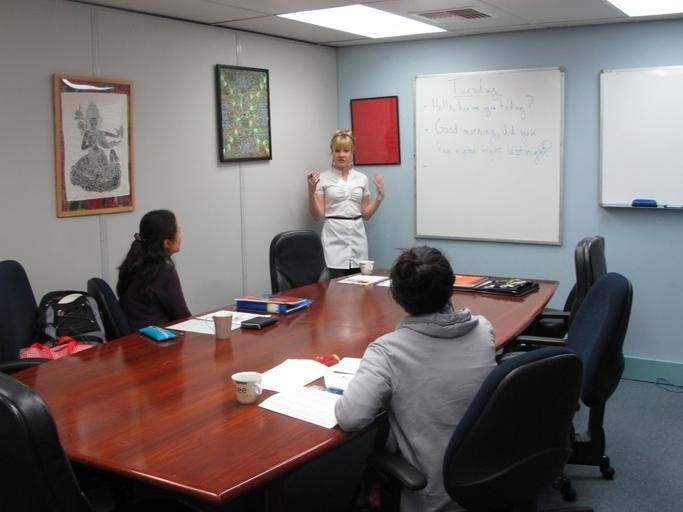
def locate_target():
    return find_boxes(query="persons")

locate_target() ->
[335,244,502,512]
[306,128,385,279]
[116,209,192,331]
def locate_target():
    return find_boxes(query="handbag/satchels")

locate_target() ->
[15,335,95,362]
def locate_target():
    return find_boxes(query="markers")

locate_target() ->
[664,205,682,208]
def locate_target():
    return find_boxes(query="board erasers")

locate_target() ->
[632,199,657,207]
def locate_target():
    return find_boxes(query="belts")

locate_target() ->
[323,215,362,221]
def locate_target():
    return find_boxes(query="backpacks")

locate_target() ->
[33,290,107,347]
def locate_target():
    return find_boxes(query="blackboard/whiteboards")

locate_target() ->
[599,65,683,210]
[415,67,565,244]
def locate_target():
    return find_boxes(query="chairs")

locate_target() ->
[364,345,594,512]
[524,234,607,411]
[0,371,114,512]
[0,259,103,375]
[495,271,634,480]
[87,277,131,342]
[269,229,330,296]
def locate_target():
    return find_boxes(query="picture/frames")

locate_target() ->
[350,95,400,166]
[216,64,278,162]
[53,71,136,218]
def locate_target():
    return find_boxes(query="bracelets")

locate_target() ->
[375,197,382,203]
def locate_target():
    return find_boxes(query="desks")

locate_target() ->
[10,267,560,512]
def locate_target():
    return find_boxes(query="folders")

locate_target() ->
[236,294,314,314]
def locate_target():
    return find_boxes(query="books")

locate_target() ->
[512,281,539,297]
[471,277,533,294]
[239,317,278,331]
[449,273,490,291]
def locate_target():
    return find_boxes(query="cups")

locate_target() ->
[360,261,375,275]
[213,315,231,339]
[231,371,263,404]
